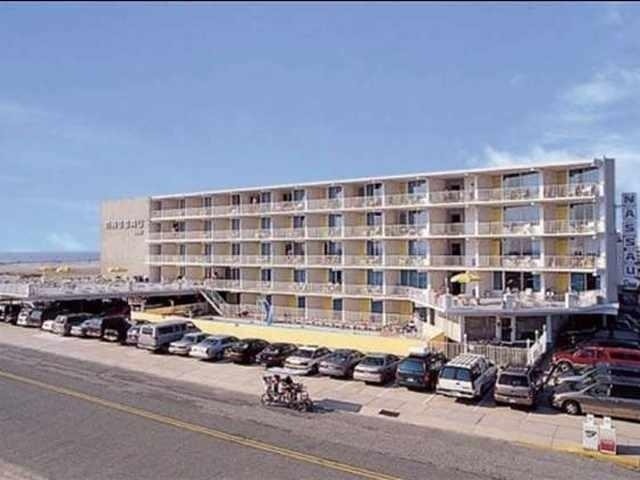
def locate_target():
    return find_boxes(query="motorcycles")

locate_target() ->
[260,375,313,414]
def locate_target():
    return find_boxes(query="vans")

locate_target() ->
[543,318,640,421]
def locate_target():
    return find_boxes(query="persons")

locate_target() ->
[171,224,177,232]
[273,374,294,395]
[287,245,292,255]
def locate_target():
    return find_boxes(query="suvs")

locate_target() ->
[435,354,496,401]
[493,365,545,406]
[396,352,448,391]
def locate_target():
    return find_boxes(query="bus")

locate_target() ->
[0,302,200,352]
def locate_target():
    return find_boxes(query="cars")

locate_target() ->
[318,349,399,384]
[170,330,332,375]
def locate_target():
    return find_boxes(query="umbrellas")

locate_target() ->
[450,271,480,294]
